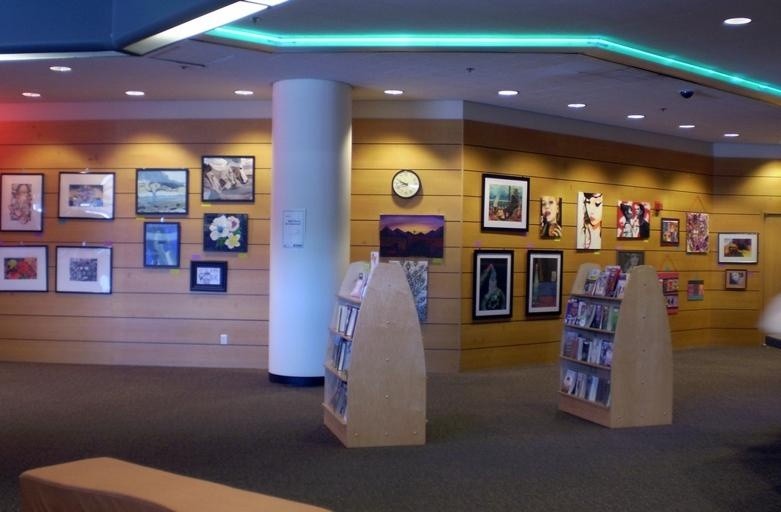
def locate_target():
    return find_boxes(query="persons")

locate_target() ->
[617,203,649,237]
[68,184,104,207]
[541,196,562,238]
[670,224,678,242]
[580,193,603,250]
[503,188,521,219]
[730,272,744,285]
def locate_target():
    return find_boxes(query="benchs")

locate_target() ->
[18,456,331,511]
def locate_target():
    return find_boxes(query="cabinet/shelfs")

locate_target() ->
[556,260,675,429]
[320,258,429,450]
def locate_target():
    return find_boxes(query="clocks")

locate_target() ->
[392,170,422,199]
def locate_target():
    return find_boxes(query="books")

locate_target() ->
[559,265,630,406]
[327,302,360,420]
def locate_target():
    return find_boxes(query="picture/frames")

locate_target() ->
[661,218,680,247]
[725,269,748,290]
[0,155,256,295]
[526,250,563,317]
[473,249,514,319]
[718,232,759,264]
[481,173,531,233]
